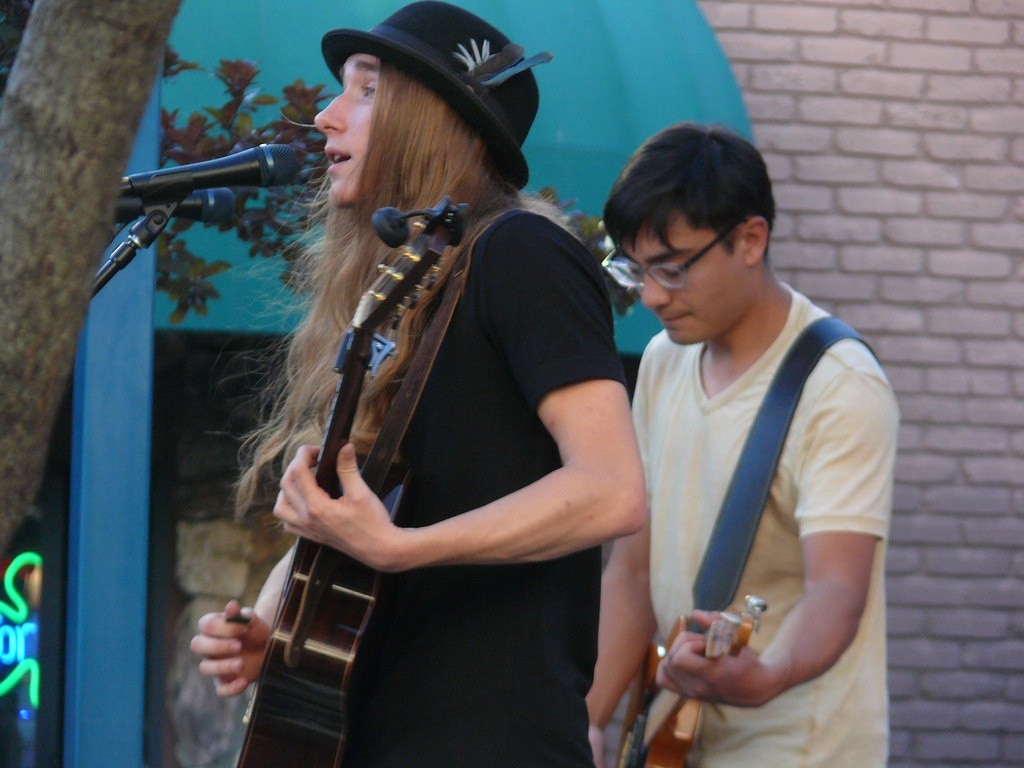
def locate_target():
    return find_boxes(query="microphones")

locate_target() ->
[114,187,236,222]
[119,142,297,196]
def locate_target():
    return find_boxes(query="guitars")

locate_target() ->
[235,194,473,768]
[613,594,770,768]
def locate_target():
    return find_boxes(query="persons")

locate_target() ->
[189,1,646,768]
[586,120,899,768]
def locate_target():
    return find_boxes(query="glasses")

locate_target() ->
[602,217,745,289]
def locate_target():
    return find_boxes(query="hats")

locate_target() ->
[321,0,551,191]
